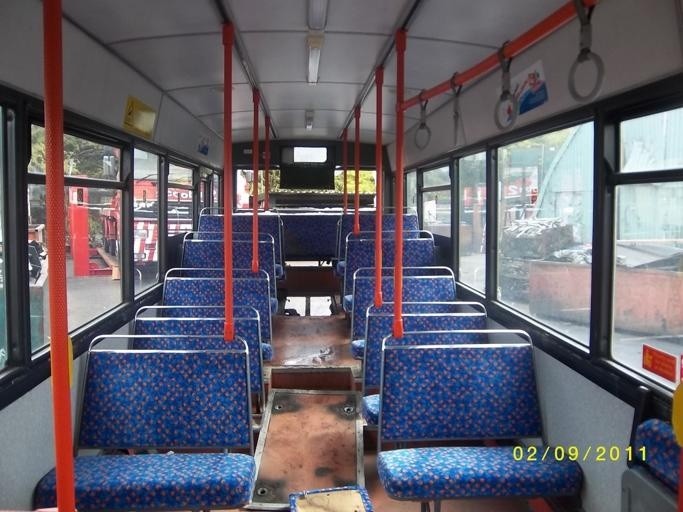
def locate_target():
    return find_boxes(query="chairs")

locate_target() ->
[333,205,586,511]
[280,213,338,262]
[26,204,285,511]
